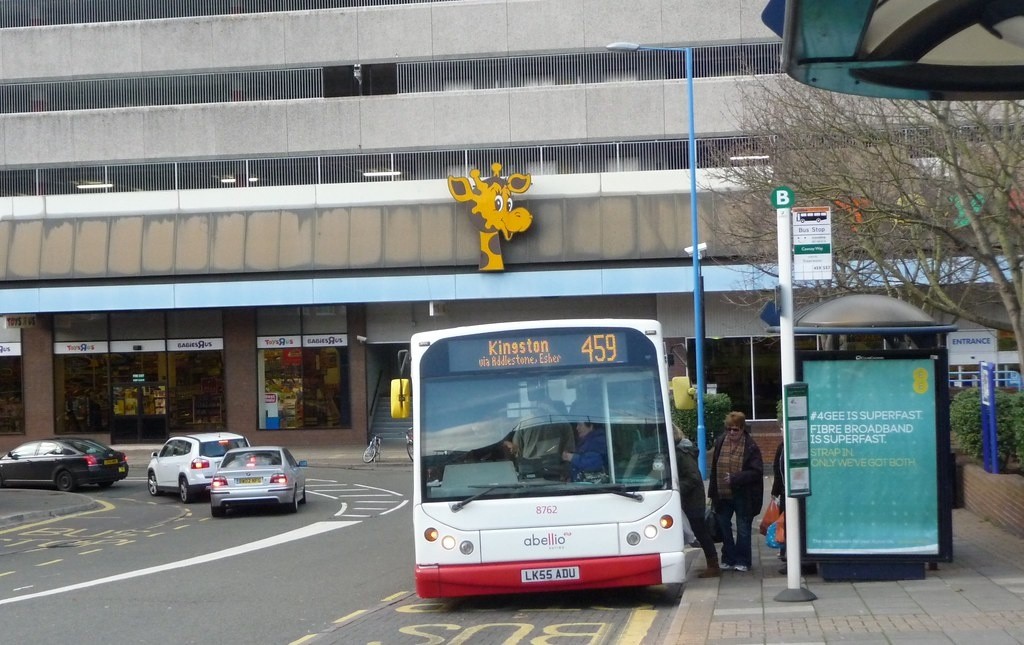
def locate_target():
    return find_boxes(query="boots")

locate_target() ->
[698,554,721,578]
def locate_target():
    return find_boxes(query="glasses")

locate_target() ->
[727,427,740,432]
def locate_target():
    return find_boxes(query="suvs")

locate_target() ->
[148,431,252,504]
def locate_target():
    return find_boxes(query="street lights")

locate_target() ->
[605,40,710,518]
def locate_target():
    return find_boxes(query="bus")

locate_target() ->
[390,318,685,599]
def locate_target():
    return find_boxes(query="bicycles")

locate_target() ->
[363,433,383,462]
[402,427,413,461]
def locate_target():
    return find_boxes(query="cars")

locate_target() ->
[0,436,129,492]
[209,445,307,517]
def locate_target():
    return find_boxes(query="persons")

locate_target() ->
[708,411,764,572]
[672,422,722,577]
[771,417,787,575]
[428,412,507,482]
[513,395,575,482]
[491,399,602,464]
[562,411,606,482]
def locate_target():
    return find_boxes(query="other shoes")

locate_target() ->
[776,553,785,559]
[778,564,787,574]
[733,565,749,572]
[720,562,731,569]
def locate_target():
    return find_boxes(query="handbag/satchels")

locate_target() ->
[765,511,786,548]
[703,496,724,543]
[758,498,779,535]
[776,512,784,543]
[579,465,608,483]
